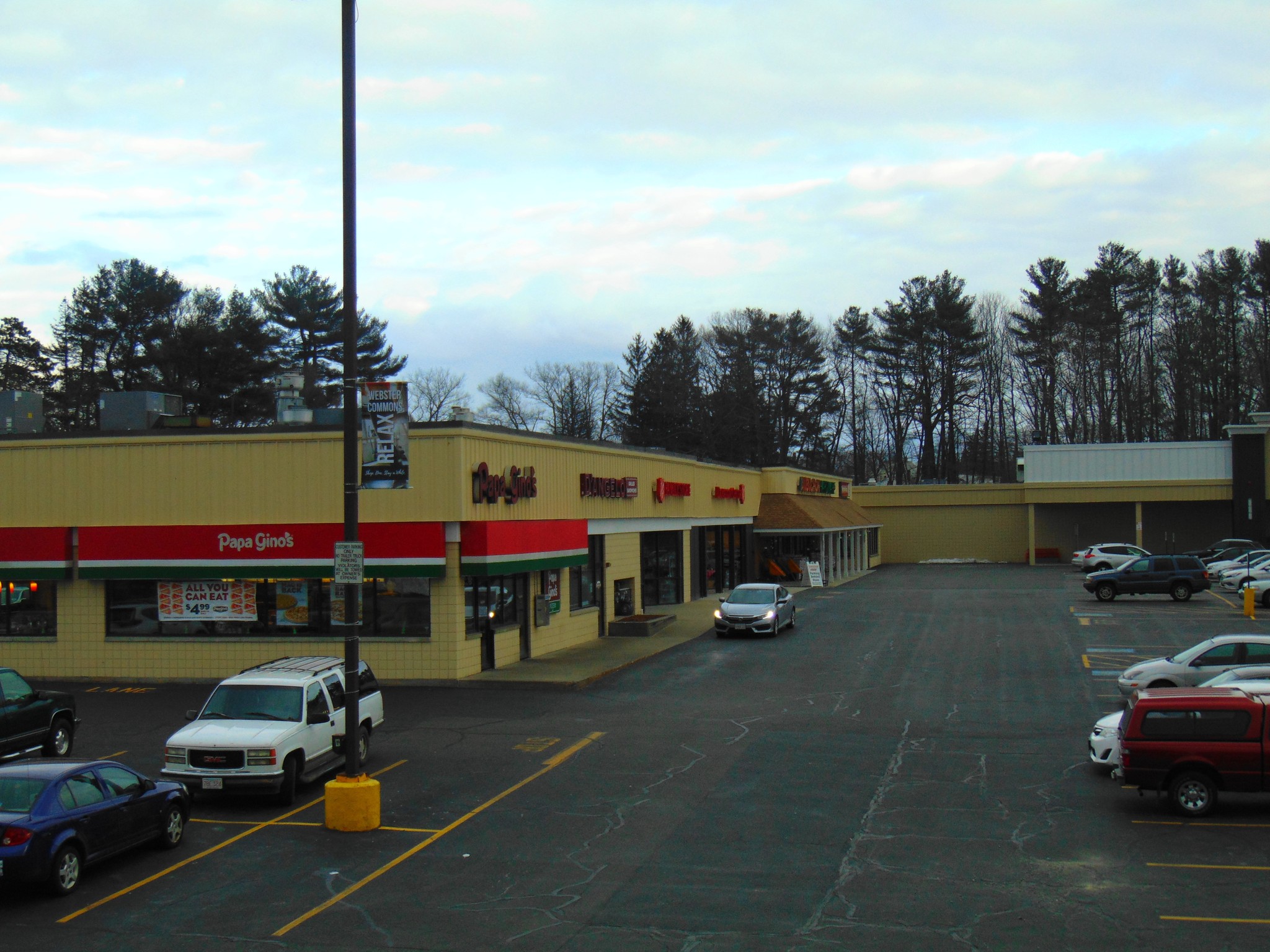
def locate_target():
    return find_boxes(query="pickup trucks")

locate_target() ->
[160,655,385,807]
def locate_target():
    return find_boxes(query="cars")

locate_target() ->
[1116,633,1270,695]
[0,667,83,762]
[1087,666,1270,771]
[1182,538,1270,610]
[1081,543,1153,574]
[1072,548,1089,568]
[0,758,192,899]
[713,583,796,638]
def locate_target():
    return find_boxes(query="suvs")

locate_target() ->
[1083,553,1212,602]
[1117,686,1270,819]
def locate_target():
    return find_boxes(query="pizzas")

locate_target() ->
[231,582,256,615]
[158,583,184,614]
[285,605,308,622]
[330,599,363,622]
[276,593,297,609]
